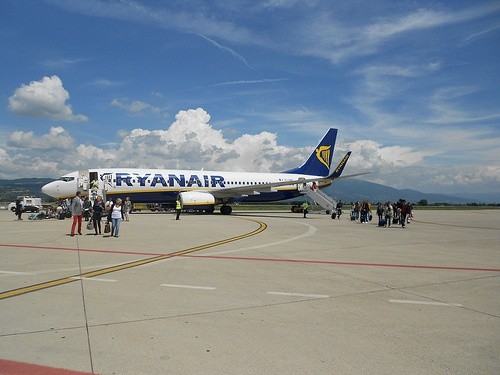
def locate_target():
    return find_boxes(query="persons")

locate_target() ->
[350,199,413,229]
[175,198,182,220]
[53,195,124,222]
[302,200,308,218]
[336,199,342,220]
[16,199,23,220]
[89,178,98,188]
[91,185,98,200]
[92,195,104,235]
[107,197,126,239]
[70,191,84,237]
[125,196,132,222]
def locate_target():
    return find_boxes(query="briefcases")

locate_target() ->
[104,222,110,233]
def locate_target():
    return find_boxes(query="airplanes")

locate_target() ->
[41,128,370,215]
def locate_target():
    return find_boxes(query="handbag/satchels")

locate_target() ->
[87,216,94,230]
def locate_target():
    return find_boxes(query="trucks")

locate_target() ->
[7,195,43,213]
